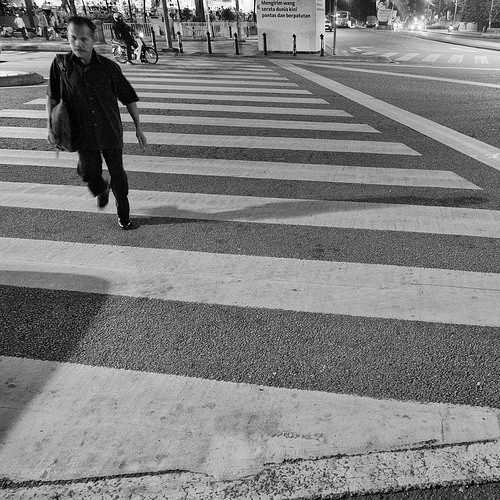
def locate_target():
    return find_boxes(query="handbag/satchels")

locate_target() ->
[50,54,78,153]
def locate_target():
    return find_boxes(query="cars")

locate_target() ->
[325,11,430,30]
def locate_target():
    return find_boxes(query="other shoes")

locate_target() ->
[98,178,110,208]
[127,61,135,65]
[117,214,130,229]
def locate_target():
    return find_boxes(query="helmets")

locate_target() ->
[113,13,122,22]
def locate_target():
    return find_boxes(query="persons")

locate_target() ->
[45,16,146,229]
[0,0,257,42]
[113,13,148,65]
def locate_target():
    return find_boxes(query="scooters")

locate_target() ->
[113,31,159,66]
